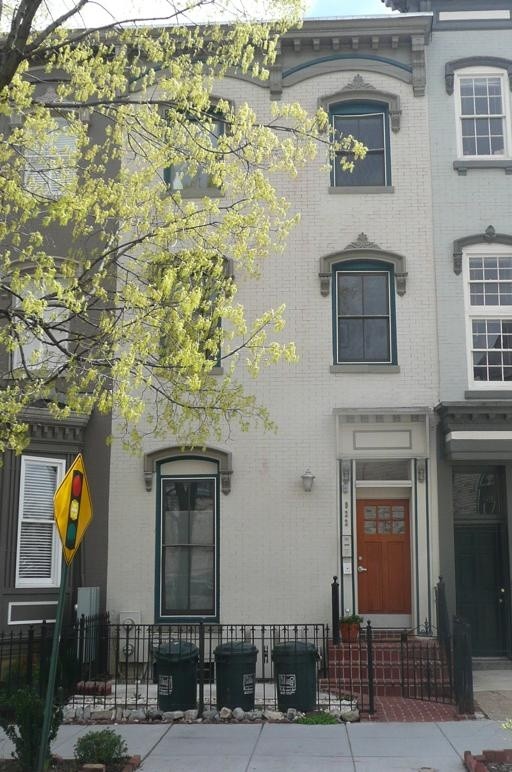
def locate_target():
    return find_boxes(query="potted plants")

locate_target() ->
[338,615,365,644]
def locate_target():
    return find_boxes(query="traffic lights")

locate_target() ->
[62,469,84,550]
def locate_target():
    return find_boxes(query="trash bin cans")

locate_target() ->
[151,641,199,711]
[271,642,318,712]
[213,641,259,712]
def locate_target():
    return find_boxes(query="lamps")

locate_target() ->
[300,469,316,492]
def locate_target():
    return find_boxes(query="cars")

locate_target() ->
[196,489,213,511]
